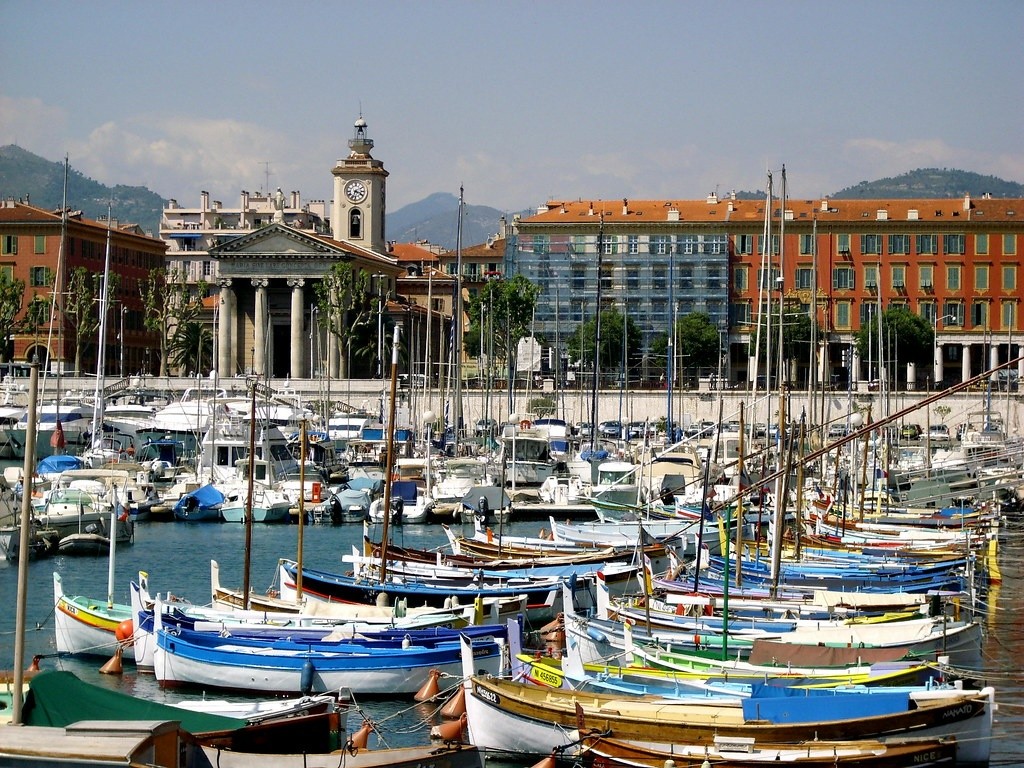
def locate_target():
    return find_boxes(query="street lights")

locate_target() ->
[310,303,319,380]
[934,312,956,390]
[120,303,127,377]
[92,275,105,372]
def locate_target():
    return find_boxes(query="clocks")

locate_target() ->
[346,182,365,202]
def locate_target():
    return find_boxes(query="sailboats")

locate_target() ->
[1,153,1024,768]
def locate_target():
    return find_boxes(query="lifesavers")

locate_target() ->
[520,420,531,430]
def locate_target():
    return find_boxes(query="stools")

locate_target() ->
[713,736,756,755]
